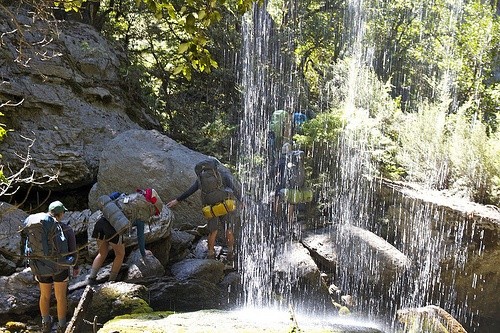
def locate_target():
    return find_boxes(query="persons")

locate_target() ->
[268,131,295,228]
[84,187,163,285]
[37,200,78,333]
[166,157,248,260]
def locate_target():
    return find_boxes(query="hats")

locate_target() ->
[49,200,68,212]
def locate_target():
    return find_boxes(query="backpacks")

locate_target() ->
[23,212,71,276]
[269,109,289,138]
[195,155,237,218]
[286,150,306,188]
[118,189,163,221]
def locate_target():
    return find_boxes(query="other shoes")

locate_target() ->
[206,251,216,258]
[88,277,98,285]
[56,325,66,333]
[42,320,53,333]
[227,254,233,262]
